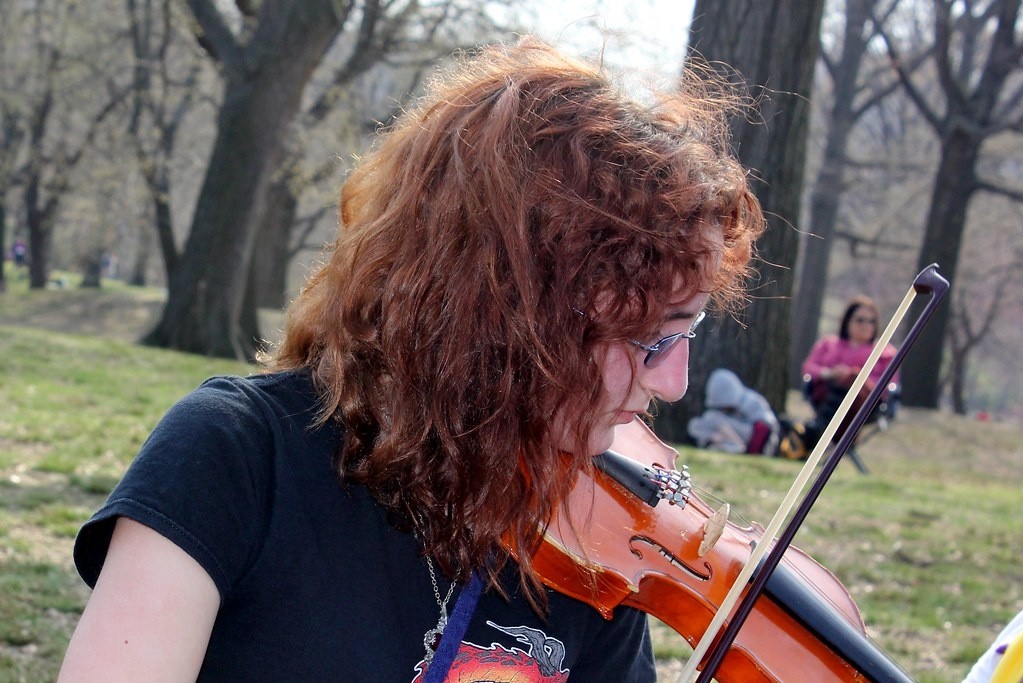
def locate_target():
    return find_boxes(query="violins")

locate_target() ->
[494,412,914,683]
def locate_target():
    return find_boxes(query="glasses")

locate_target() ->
[572,305,706,370]
[854,316,877,325]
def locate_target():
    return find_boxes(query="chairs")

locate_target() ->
[801,384,891,474]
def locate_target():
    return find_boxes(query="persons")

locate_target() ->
[55,35,791,683]
[802,299,904,462]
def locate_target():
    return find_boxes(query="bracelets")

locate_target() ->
[821,366,835,381]
